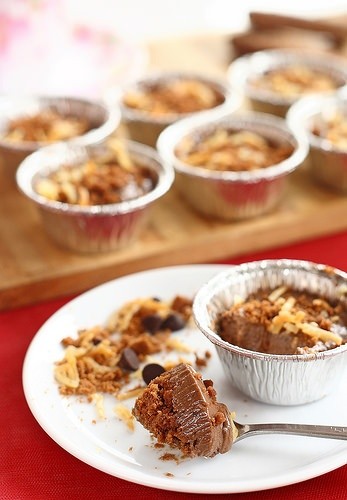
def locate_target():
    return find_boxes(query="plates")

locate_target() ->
[20,264,347,495]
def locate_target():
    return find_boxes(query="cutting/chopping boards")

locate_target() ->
[0,13,346,312]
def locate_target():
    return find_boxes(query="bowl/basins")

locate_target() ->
[227,46,347,118]
[0,90,122,161]
[14,138,176,254]
[192,259,347,407]
[284,92,347,193]
[155,108,311,220]
[117,71,241,147]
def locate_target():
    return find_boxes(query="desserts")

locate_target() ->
[217,285,347,355]
[135,362,238,462]
[1,49,347,253]
